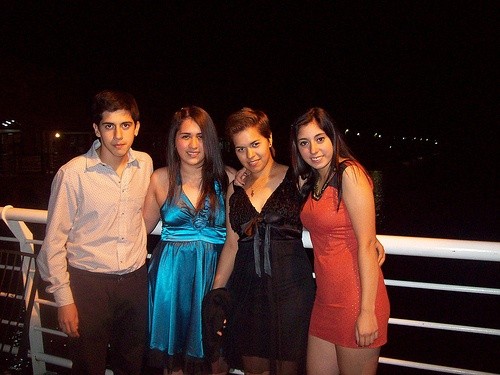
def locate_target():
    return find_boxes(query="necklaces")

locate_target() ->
[251,162,276,197]
[315,167,332,200]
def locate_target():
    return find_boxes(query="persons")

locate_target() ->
[212,107,386,375]
[36,90,154,375]
[140,107,238,375]
[234,106,392,375]
